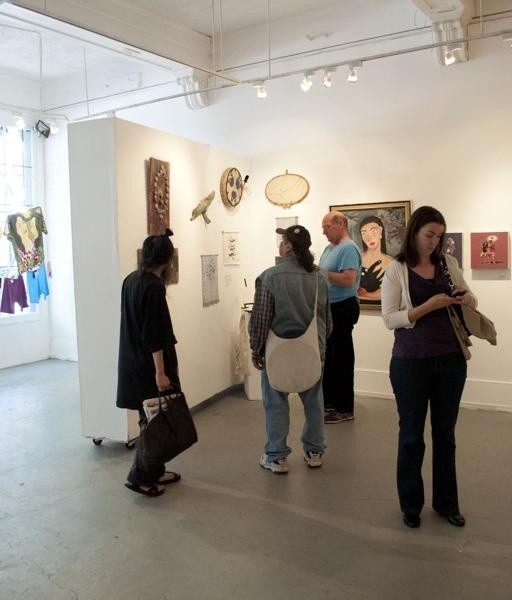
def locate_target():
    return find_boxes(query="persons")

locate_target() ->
[380,206,482,528]
[248,224,332,476]
[353,213,397,305]
[317,210,363,428]
[117,226,181,498]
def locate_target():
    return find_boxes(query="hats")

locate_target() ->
[276,225,312,247]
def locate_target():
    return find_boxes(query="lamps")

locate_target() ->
[246,31,465,100]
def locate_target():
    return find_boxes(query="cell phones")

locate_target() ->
[450,289,465,297]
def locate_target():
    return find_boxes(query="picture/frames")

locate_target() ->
[328,199,414,312]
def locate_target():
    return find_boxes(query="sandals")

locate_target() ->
[157,472,181,484]
[123,482,163,497]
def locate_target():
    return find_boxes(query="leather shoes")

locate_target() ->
[403,512,421,529]
[449,514,465,526]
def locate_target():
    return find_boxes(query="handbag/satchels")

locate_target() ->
[138,388,198,473]
[264,317,321,393]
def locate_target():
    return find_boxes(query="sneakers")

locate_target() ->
[303,449,323,468]
[323,411,354,423]
[258,453,290,474]
